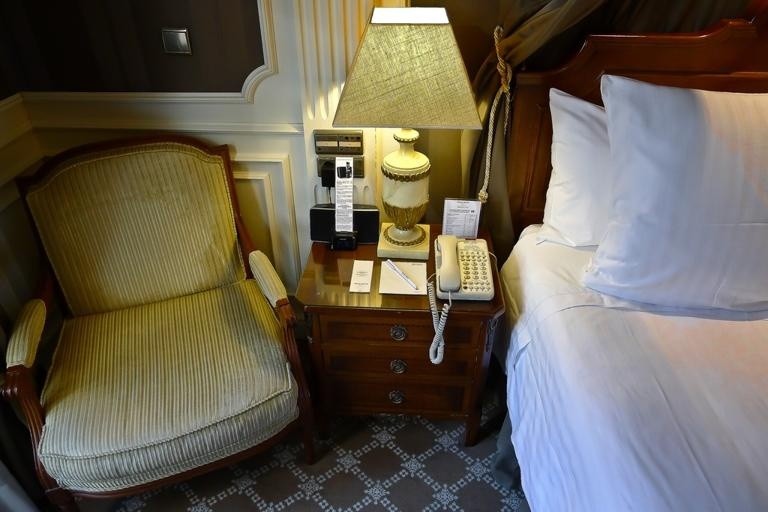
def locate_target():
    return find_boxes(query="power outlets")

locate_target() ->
[313,129,365,179]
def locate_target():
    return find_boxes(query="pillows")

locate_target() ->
[536,87,608,247]
[580,75,768,313]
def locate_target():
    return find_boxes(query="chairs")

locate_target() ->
[0,132,326,511]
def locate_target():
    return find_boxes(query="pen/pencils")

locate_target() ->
[386,258,417,290]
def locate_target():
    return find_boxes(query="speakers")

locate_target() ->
[309,203,379,243]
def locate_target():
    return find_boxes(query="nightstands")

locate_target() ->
[293,223,506,447]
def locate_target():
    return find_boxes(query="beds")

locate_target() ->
[494,0,768,512]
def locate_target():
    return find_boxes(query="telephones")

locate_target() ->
[434,235,495,301]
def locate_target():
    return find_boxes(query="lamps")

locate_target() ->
[332,7,484,260]
